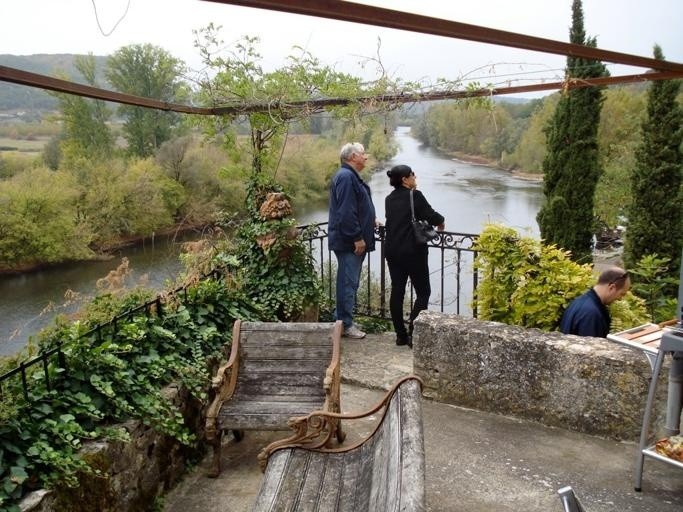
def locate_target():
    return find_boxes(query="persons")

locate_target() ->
[560,267,631,338]
[384,165,445,348]
[328,143,384,340]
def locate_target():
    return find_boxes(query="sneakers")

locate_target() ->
[344,326,366,339]
[396,334,408,345]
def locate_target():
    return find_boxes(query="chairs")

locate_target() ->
[252,376,426,512]
[205,319,346,478]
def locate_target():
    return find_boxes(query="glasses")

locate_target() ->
[609,272,630,286]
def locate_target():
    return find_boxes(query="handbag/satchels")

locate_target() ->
[409,190,439,245]
